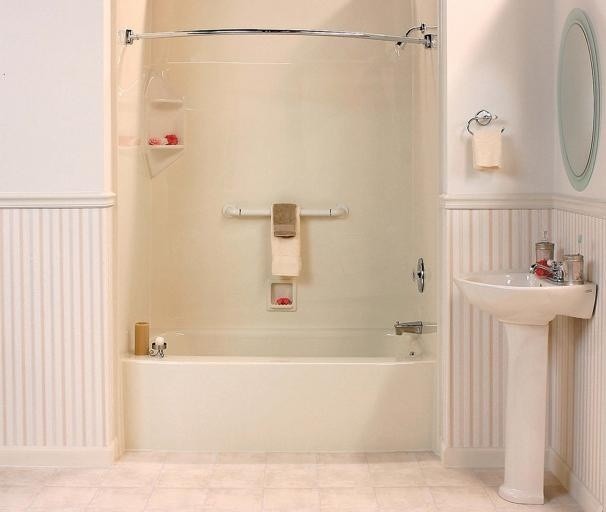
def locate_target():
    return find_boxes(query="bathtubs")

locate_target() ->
[121,326,436,455]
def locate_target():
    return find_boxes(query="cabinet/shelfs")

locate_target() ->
[132,96,185,177]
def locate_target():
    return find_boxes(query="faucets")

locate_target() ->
[529,259,565,283]
[393,319,423,336]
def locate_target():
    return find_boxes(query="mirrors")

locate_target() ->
[554,7,600,192]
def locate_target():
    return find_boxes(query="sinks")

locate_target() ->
[452,272,596,322]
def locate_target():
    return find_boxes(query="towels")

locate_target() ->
[470,128,502,173]
[268,200,301,281]
